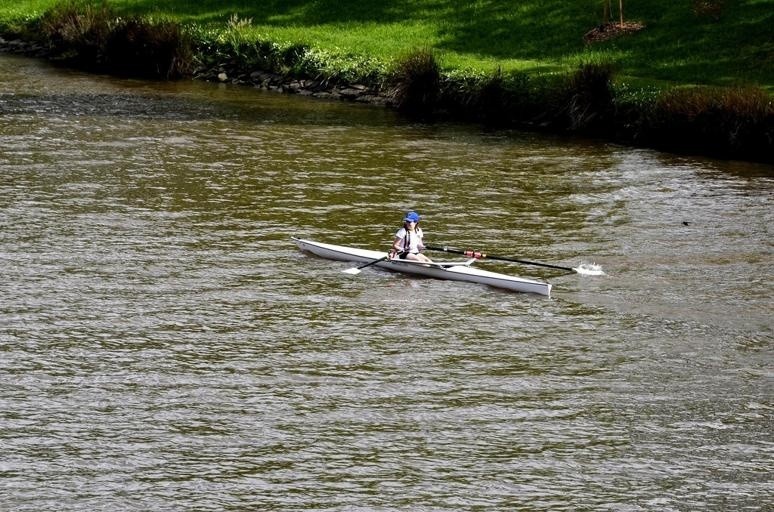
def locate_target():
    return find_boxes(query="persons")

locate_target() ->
[393,212,433,267]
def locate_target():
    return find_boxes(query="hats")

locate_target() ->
[404,212,420,222]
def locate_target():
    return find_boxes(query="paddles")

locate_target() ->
[341,247,410,274]
[423,245,605,275]
